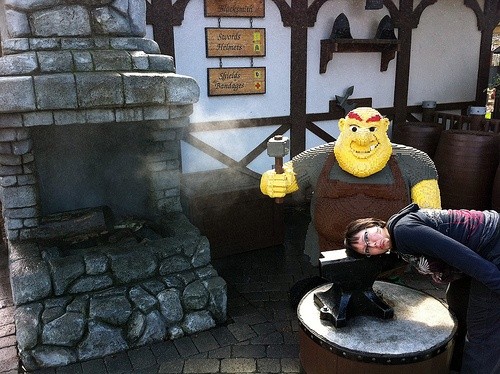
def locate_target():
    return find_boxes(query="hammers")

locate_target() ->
[266,134,290,204]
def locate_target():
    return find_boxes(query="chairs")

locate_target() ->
[434,112,481,132]
[480,119,500,132]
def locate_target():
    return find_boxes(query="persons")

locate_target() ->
[257,106,445,273]
[342,199,500,317]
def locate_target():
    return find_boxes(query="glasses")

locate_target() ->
[364,227,372,258]
